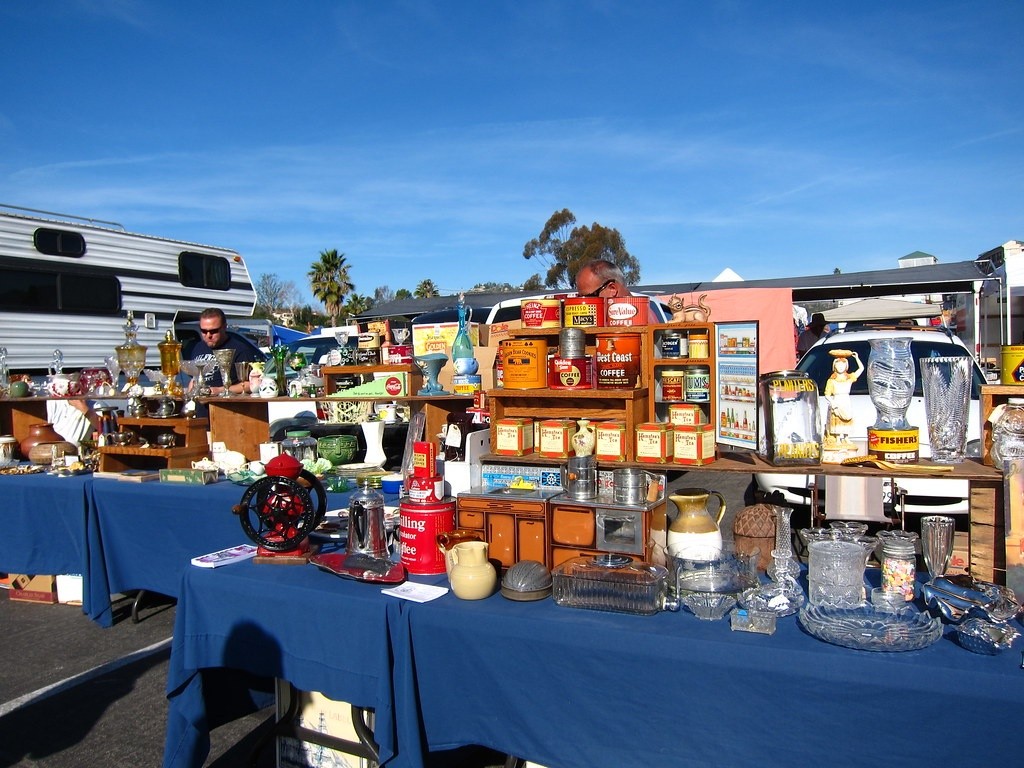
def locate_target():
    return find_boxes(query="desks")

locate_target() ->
[1,452,1024,768]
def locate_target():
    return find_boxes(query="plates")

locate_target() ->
[922,574,1023,623]
[297,506,400,540]
[799,597,945,651]
[735,586,804,618]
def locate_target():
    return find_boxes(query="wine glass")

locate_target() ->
[921,516,956,592]
[212,349,235,398]
[234,362,249,396]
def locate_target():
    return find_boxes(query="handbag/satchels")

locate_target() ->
[52,405,95,447]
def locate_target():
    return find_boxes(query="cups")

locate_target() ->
[919,356,973,463]
[0,434,16,462]
[560,455,597,503]
[334,331,350,347]
[612,467,660,505]
[866,338,916,430]
[391,328,410,346]
[559,328,585,359]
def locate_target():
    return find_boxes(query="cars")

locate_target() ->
[405,308,492,344]
[757,327,989,460]
[263,334,362,382]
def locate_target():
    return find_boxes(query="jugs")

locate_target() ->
[667,487,726,577]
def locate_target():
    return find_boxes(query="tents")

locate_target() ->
[793,305,808,327]
[811,298,947,335]
[976,252,1024,346]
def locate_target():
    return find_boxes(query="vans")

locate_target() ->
[485,291,673,324]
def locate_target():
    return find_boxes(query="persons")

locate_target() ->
[187,308,254,397]
[793,313,832,360]
[52,400,119,444]
[576,260,659,325]
[822,349,864,463]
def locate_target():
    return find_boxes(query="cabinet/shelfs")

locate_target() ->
[489,319,718,455]
[2,362,479,473]
[549,493,666,584]
[454,492,560,574]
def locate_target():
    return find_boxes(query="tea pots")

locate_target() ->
[346,479,390,559]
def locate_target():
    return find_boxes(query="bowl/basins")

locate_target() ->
[663,539,761,591]
[327,476,348,492]
[682,593,737,620]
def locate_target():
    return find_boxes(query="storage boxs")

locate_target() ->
[493,405,716,467]
[4,571,57,604]
[411,320,527,394]
[274,676,381,768]
[57,574,136,606]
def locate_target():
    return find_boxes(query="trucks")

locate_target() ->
[0,204,257,384]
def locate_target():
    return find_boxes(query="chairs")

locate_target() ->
[808,473,907,529]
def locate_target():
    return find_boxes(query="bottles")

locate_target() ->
[449,540,497,600]
[767,506,803,596]
[452,294,474,361]
[992,398,1024,469]
[281,430,318,462]
[571,420,595,457]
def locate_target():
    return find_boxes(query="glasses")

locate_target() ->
[575,279,615,298]
[201,326,223,334]
